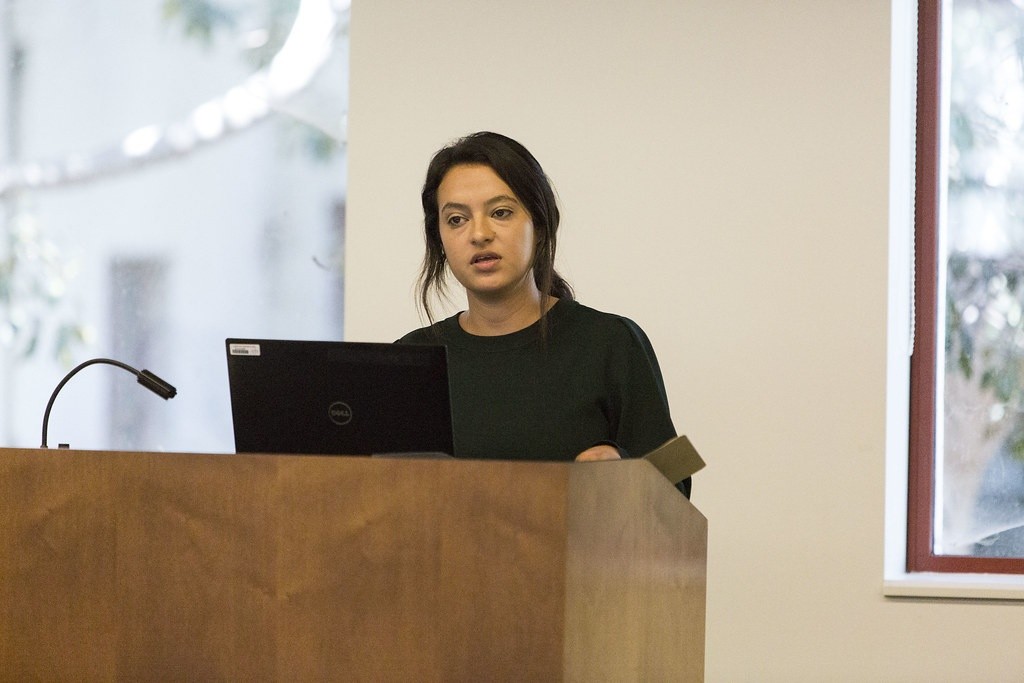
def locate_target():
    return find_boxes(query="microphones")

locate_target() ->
[38,357,176,450]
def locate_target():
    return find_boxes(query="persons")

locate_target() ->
[392,132,694,500]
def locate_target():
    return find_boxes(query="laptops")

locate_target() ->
[226,338,455,460]
